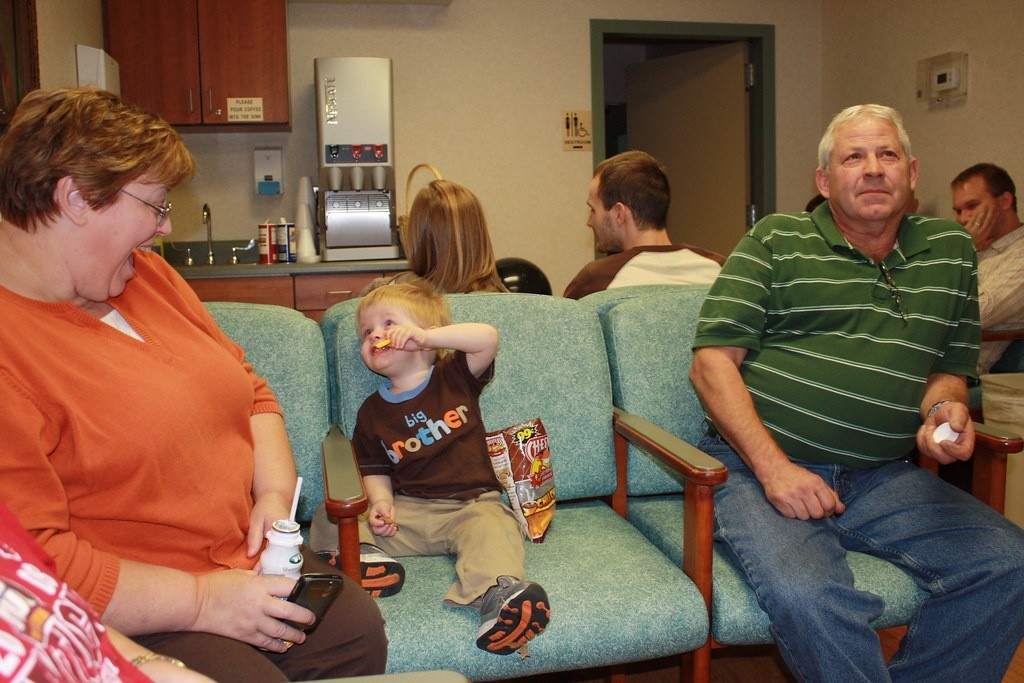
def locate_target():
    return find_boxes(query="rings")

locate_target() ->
[974,222,981,226]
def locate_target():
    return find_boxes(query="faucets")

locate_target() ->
[201,203,217,265]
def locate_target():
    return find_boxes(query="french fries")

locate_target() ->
[375,339,391,348]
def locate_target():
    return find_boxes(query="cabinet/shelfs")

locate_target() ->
[103,0,293,134]
[184,271,409,326]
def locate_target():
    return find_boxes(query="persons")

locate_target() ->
[563,151,727,301]
[0,87,388,683]
[805,163,1024,374]
[358,180,513,297]
[309,284,551,655]
[689,104,1024,683]
[0,500,218,683]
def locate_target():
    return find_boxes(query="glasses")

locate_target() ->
[879,260,910,328]
[115,187,172,228]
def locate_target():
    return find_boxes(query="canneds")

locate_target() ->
[258,217,296,264]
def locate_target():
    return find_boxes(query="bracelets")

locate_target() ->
[924,400,951,419]
[130,652,188,669]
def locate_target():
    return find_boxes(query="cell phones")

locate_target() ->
[277,572,343,635]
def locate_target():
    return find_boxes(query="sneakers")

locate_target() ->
[475,577,550,657]
[318,543,405,596]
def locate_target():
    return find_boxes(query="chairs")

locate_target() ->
[201,295,331,552]
[321,293,729,683]
[605,289,1024,683]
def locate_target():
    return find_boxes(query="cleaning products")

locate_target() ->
[135,234,164,258]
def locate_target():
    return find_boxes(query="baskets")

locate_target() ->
[398,164,442,261]
